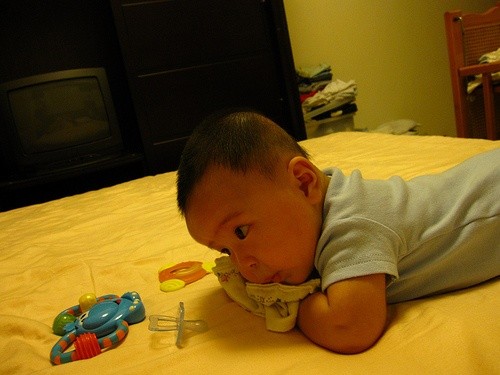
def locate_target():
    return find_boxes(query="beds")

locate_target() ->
[0,130,500,375]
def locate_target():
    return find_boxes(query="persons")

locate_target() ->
[177,110,500,354]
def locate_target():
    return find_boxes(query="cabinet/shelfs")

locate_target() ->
[0,0,308,213]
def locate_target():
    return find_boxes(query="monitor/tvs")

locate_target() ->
[0,67,124,176]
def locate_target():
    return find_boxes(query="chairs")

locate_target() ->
[444,0,500,141]
